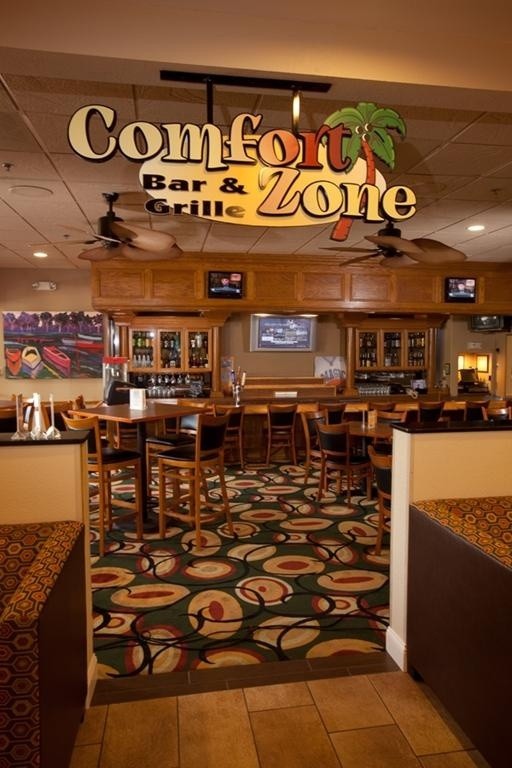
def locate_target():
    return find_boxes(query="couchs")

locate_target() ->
[0,520,86,768]
[408,498,512,768]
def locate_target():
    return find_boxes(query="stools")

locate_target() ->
[63,395,236,553]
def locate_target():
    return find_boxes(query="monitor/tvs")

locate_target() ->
[471,316,503,332]
[208,271,242,299]
[250,313,319,352]
[444,277,476,303]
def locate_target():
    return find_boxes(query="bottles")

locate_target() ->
[384,332,401,367]
[359,333,377,367]
[133,331,153,369]
[160,332,181,368]
[408,332,425,366]
[146,372,191,399]
[189,331,209,368]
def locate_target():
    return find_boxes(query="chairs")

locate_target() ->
[299,407,512,557]
[214,399,490,466]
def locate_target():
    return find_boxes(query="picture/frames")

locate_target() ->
[458,355,465,371]
[476,355,489,373]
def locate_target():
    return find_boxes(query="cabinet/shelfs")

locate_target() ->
[355,329,429,370]
[127,317,213,374]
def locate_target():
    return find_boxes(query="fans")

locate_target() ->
[26,192,184,262]
[319,221,467,270]
